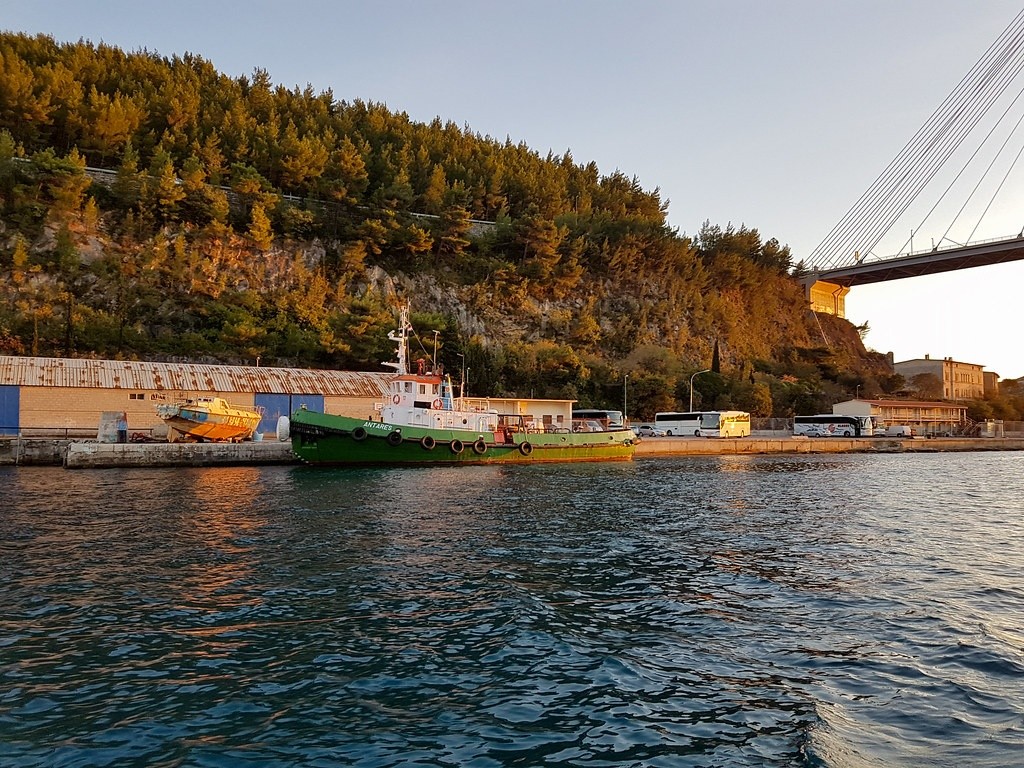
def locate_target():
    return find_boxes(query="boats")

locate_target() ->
[155,392,266,444]
[279,294,645,469]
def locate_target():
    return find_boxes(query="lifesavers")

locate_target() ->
[472,440,488,455]
[421,435,436,450]
[450,439,464,455]
[388,431,403,446]
[351,426,368,441]
[519,441,534,456]
[393,394,400,404]
[433,399,443,409]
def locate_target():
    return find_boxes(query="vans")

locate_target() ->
[884,425,912,438]
[571,418,603,432]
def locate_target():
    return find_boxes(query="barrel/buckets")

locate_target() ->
[117,430,126,442]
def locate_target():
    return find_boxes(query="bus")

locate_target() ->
[654,409,713,438]
[572,409,624,433]
[697,410,751,440]
[794,414,885,438]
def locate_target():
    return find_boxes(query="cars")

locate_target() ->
[629,425,640,438]
[637,424,667,438]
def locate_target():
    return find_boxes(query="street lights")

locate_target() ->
[457,352,465,384]
[857,385,861,399]
[432,329,441,370]
[690,370,711,412]
[625,374,629,425]
[466,367,471,397]
